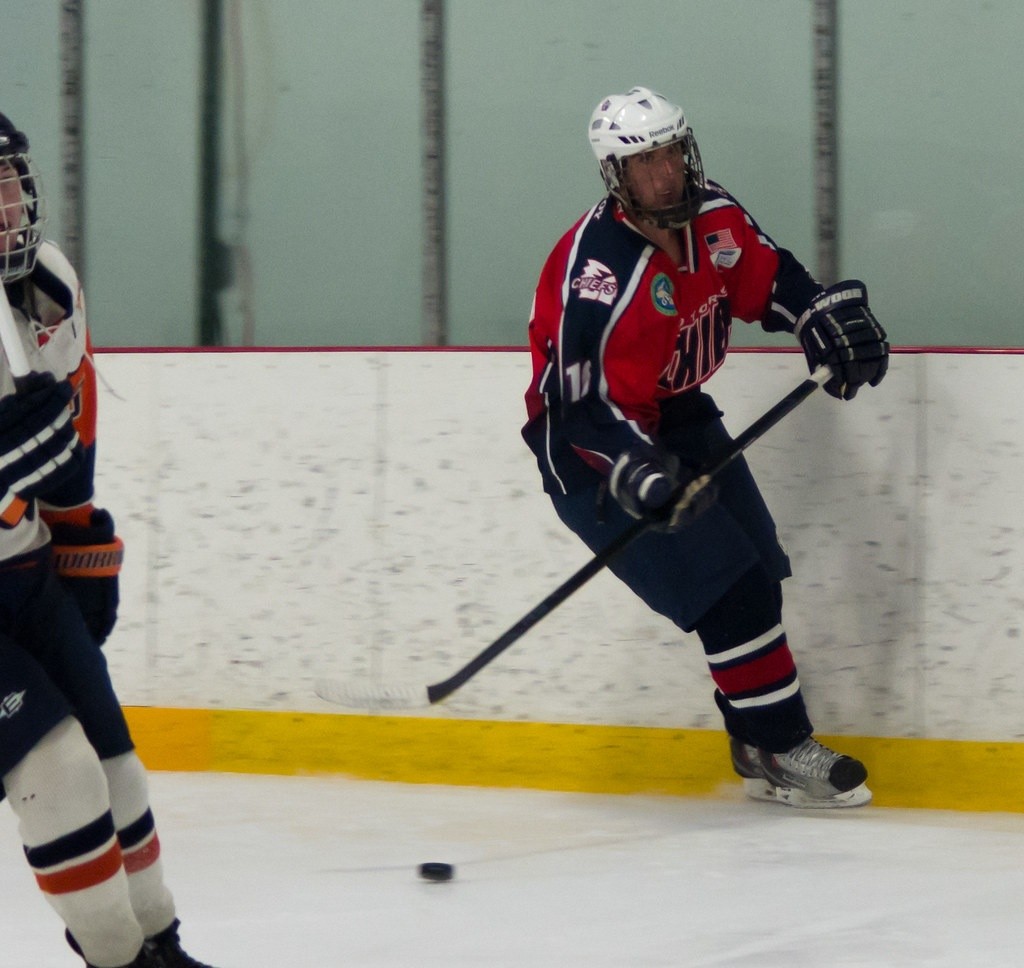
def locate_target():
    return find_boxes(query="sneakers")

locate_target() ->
[713,689,872,808]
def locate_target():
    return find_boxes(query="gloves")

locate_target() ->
[611,445,718,532]
[791,279,892,398]
[49,507,120,646]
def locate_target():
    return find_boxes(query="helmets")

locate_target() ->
[0,111,46,280]
[586,86,704,229]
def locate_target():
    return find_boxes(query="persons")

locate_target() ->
[520,86,891,810]
[0,112,215,968]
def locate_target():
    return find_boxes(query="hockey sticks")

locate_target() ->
[312,359,835,711]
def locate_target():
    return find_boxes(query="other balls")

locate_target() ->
[418,862,452,884]
